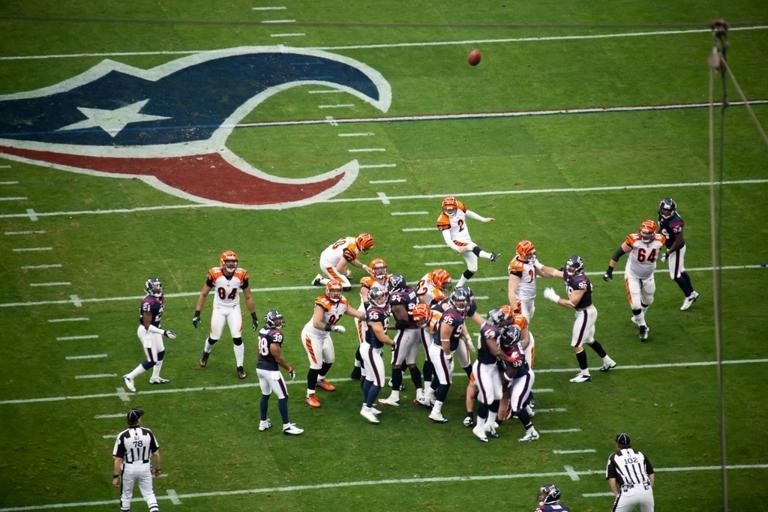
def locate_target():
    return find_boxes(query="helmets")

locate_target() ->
[325,279,343,302]
[219,250,238,277]
[411,268,473,328]
[639,219,656,239]
[356,233,373,251]
[565,255,584,276]
[658,197,677,220]
[368,259,406,309]
[143,277,163,298]
[515,240,536,262]
[540,484,560,504]
[492,305,528,348]
[263,309,285,329]
[441,196,458,216]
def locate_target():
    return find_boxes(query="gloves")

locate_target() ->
[289,370,295,380]
[390,342,400,351]
[331,324,345,334]
[193,316,200,328]
[603,270,612,281]
[164,329,177,339]
[462,416,473,427]
[544,287,560,304]
[252,320,258,331]
[661,253,668,262]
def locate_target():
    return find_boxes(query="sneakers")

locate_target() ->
[415,388,448,422]
[569,374,591,383]
[258,421,272,431]
[351,366,405,424]
[631,315,649,342]
[680,290,700,311]
[600,359,616,371]
[311,274,323,285]
[491,251,503,262]
[200,352,208,367]
[472,425,500,443]
[238,367,247,379]
[149,377,169,384]
[122,375,136,392]
[519,399,540,442]
[305,393,321,407]
[317,379,336,391]
[283,425,304,435]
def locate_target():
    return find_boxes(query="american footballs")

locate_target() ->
[469,49,481,65]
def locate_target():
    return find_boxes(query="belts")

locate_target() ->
[127,460,148,464]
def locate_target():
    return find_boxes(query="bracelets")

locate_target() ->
[155,468,161,473]
[112,474,120,478]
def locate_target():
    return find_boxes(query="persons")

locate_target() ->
[300,255,539,444]
[506,240,564,325]
[604,433,655,512]
[534,483,570,512]
[543,253,617,383]
[255,309,305,435]
[120,277,176,392]
[112,408,161,511]
[310,232,375,292]
[602,219,666,342]
[191,250,259,380]
[657,197,700,312]
[435,195,497,288]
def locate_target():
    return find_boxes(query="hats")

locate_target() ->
[127,409,144,421]
[615,433,630,445]
